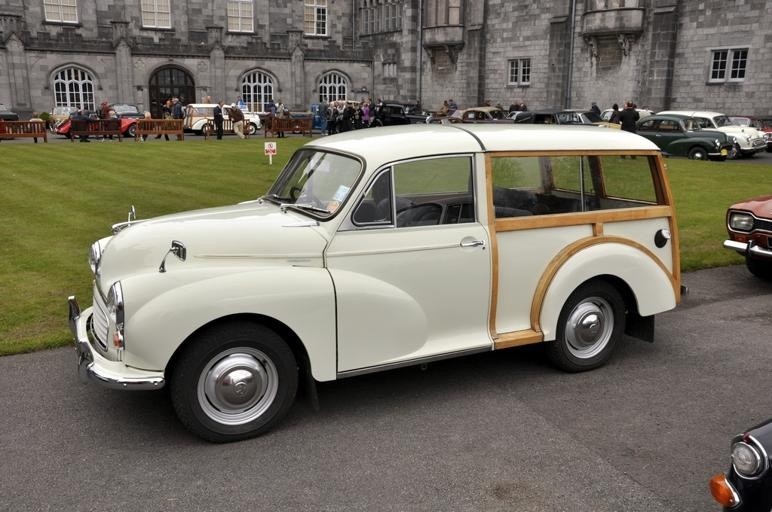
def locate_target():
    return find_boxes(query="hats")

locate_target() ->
[230,103,236,108]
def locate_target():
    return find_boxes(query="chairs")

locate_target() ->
[373,186,582,228]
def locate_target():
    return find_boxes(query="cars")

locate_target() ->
[48,102,263,139]
[68,121,681,443]
[708,418,772,512]
[724,193,772,289]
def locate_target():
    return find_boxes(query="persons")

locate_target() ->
[483,98,528,116]
[71,102,123,141]
[268,100,285,138]
[440,99,457,116]
[26,111,48,143]
[139,98,185,141]
[214,96,246,139]
[319,97,384,136]
[590,100,641,160]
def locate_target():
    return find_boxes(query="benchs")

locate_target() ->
[263,116,314,139]
[203,119,251,140]
[134,118,184,142]
[68,118,123,142]
[1,120,48,142]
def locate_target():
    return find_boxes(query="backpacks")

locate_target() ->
[181,108,186,118]
